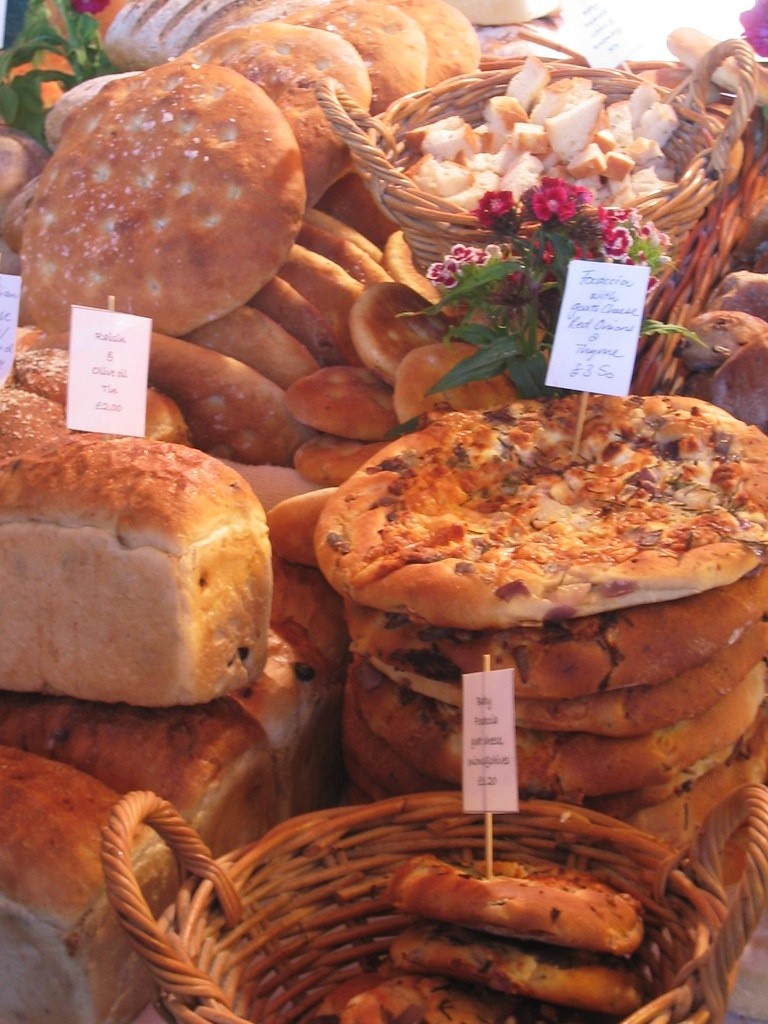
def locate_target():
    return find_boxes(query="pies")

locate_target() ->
[313,392,768,1023]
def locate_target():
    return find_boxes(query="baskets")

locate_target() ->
[95,784,768,1024]
[632,157,768,403]
[310,39,758,313]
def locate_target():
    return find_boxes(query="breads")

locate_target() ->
[637,25,768,107]
[0,0,681,1023]
[676,197,768,435]
